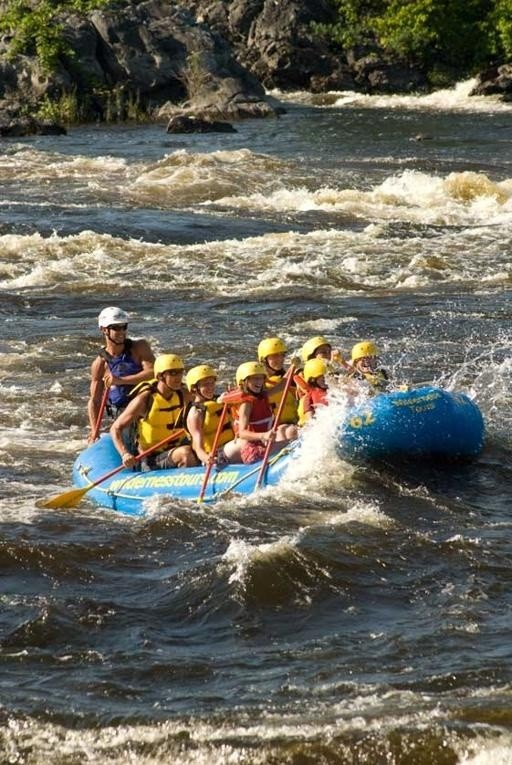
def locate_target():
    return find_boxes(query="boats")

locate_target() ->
[70,379,489,511]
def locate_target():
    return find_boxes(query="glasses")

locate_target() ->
[106,324,128,331]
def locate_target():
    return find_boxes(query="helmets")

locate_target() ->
[351,341,378,364]
[257,338,288,366]
[302,336,331,363]
[304,357,334,384]
[98,306,131,330]
[154,354,186,378]
[185,364,218,393]
[236,362,269,387]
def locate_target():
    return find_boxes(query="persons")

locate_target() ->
[109,353,199,471]
[348,340,410,396]
[230,361,301,464]
[184,364,249,466]
[303,334,347,369]
[85,306,156,445]
[298,356,353,428]
[256,334,298,428]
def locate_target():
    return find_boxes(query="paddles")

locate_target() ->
[44,430,186,510]
